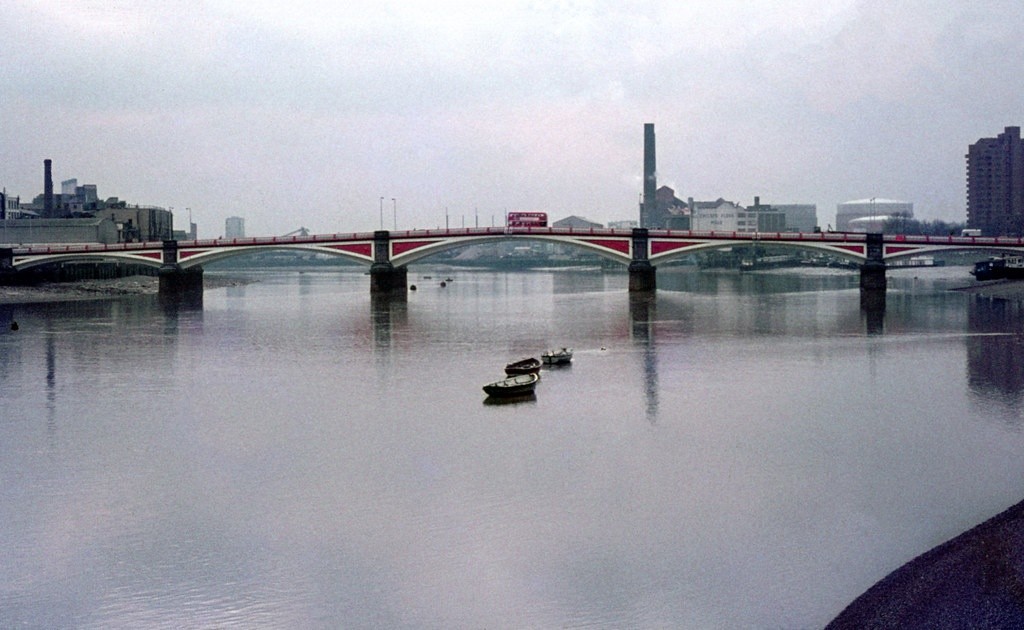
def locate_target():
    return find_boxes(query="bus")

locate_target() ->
[508,212,547,227]
[961,229,981,237]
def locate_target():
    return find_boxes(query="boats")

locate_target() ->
[483,373,538,396]
[445,279,453,281]
[969,254,1024,281]
[541,348,573,365]
[505,358,542,376]
